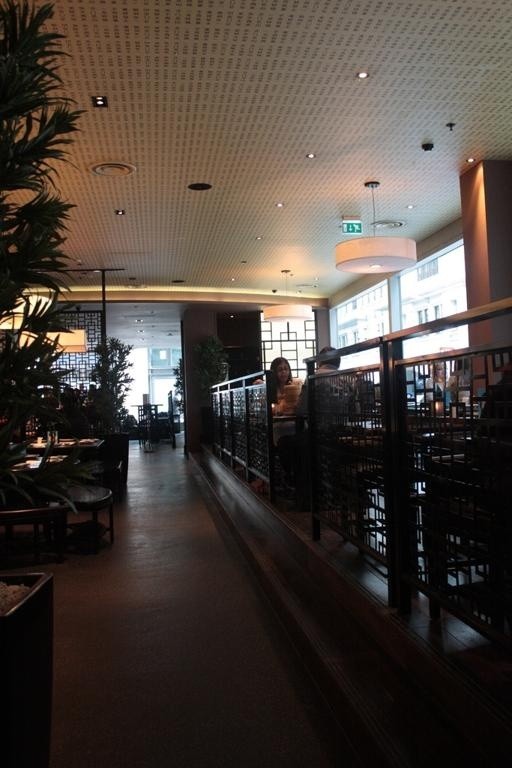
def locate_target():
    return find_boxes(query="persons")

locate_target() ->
[269,357,300,414]
[277,347,364,511]
[424,359,450,411]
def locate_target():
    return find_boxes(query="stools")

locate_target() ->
[59,486,115,555]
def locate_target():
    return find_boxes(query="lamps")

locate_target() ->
[0,293,88,354]
[335,178,418,275]
